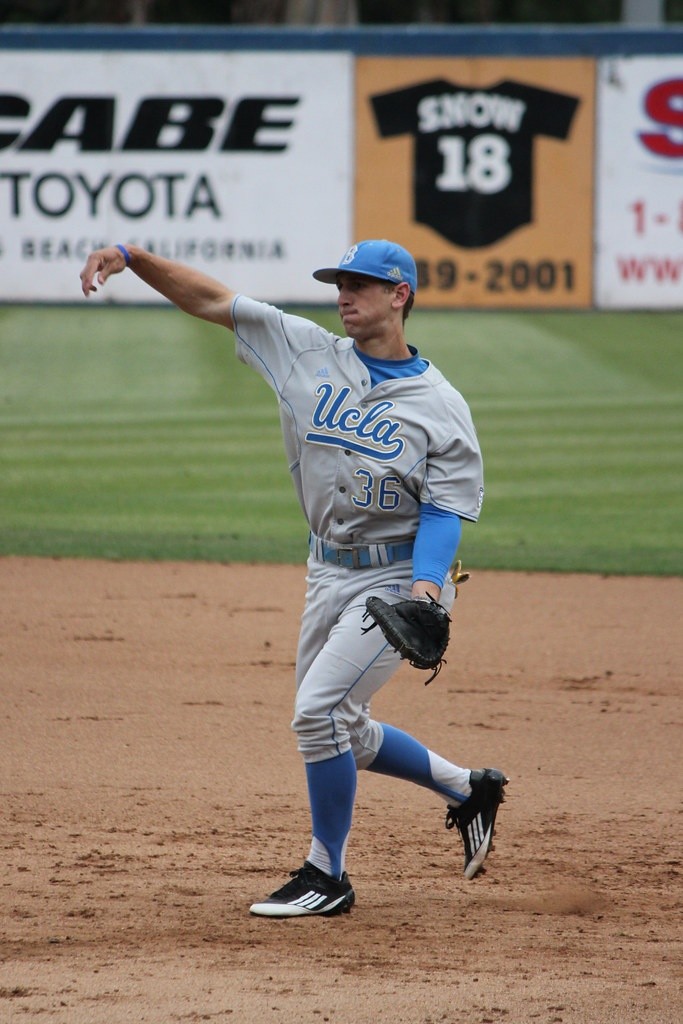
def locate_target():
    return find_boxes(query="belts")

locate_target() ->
[310,534,414,568]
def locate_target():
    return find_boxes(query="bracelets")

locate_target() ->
[115,244,130,264]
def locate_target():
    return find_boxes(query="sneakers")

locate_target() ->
[445,769,509,881]
[249,860,355,918]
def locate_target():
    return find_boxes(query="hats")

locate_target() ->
[312,239,417,295]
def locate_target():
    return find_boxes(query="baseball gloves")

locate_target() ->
[357,591,456,687]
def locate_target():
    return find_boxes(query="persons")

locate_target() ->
[80,238,510,919]
